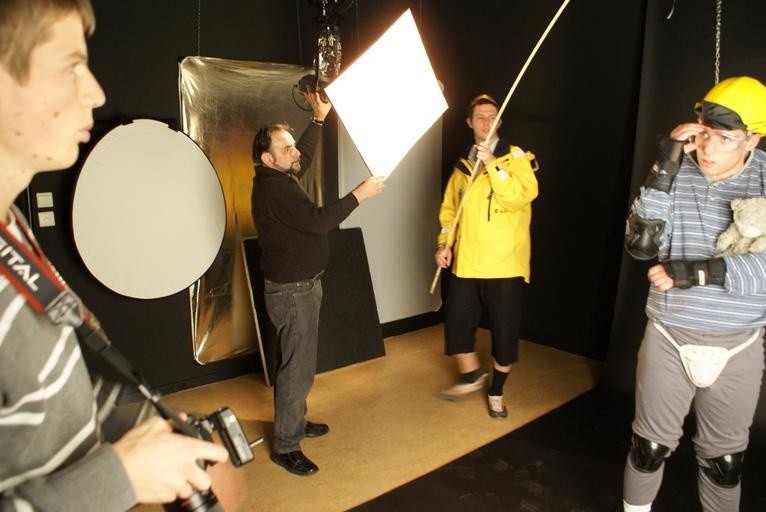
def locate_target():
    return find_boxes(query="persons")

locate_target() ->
[623,75,764,511]
[250,84,388,475]
[0,1,230,511]
[435,94,541,419]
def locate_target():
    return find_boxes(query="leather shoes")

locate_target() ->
[305,420,330,437]
[271,449,318,475]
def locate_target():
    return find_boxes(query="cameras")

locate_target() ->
[164,408,264,512]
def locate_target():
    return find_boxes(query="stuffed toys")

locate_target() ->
[715,195,765,257]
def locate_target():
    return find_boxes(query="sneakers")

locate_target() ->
[488,392,506,419]
[444,373,489,395]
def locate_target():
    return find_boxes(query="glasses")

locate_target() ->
[694,128,748,148]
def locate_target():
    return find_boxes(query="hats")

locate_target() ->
[693,76,766,137]
